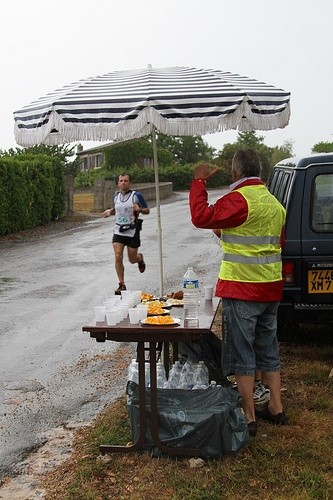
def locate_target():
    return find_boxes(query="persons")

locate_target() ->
[189,148,288,437]
[103,173,149,295]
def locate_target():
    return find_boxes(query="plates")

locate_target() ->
[167,299,183,305]
[147,309,170,314]
[145,302,172,307]
[141,295,157,300]
[141,316,180,325]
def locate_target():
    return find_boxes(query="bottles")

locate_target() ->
[126,358,223,405]
[182,267,199,319]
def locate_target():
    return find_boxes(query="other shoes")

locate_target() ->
[115,285,126,295]
[255,403,288,425]
[247,421,258,436]
[139,253,145,273]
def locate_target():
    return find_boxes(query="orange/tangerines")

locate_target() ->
[141,293,164,314]
[145,314,173,325]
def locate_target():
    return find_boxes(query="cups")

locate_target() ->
[204,287,213,300]
[93,290,142,326]
[128,305,148,324]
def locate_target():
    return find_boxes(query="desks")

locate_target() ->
[82,296,221,455]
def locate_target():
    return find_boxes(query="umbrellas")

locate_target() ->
[12,63,291,296]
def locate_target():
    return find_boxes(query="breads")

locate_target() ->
[168,291,184,300]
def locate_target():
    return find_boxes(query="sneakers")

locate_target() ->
[253,384,270,405]
[232,379,260,390]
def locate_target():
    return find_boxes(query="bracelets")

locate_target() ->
[139,207,144,213]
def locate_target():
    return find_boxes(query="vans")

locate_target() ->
[266,152,333,340]
